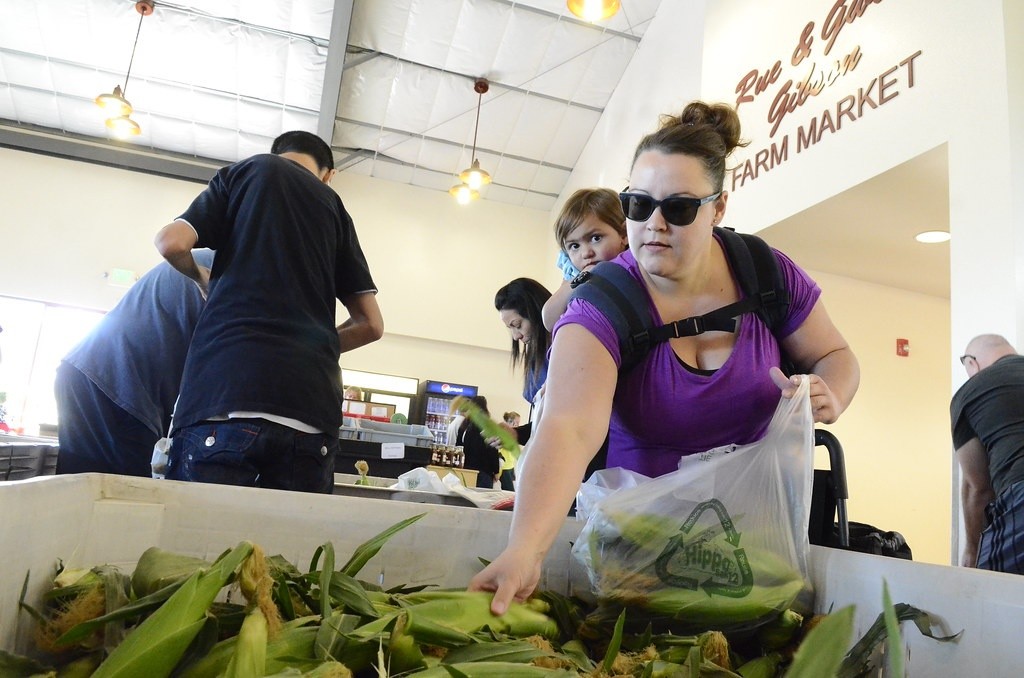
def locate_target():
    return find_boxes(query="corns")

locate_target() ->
[454,397,521,459]
[355,461,368,485]
[0,509,859,678]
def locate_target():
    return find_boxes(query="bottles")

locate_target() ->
[424,397,465,468]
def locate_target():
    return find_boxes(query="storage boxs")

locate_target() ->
[0,472,1024,678]
[338,416,435,447]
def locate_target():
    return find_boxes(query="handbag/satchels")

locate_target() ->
[572,375,815,621]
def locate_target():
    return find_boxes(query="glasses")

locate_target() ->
[960,355,976,365]
[619,186,721,226]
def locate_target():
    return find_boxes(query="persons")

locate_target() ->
[155,130,384,494]
[55,249,215,478]
[468,99,860,616]
[481,278,609,517]
[542,188,628,332]
[344,386,361,401]
[446,397,521,492]
[949,334,1024,574]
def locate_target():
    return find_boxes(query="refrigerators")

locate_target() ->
[420,381,478,444]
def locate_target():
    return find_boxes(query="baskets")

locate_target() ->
[427,464,479,488]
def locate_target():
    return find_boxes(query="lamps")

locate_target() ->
[449,78,494,209]
[94,0,156,139]
